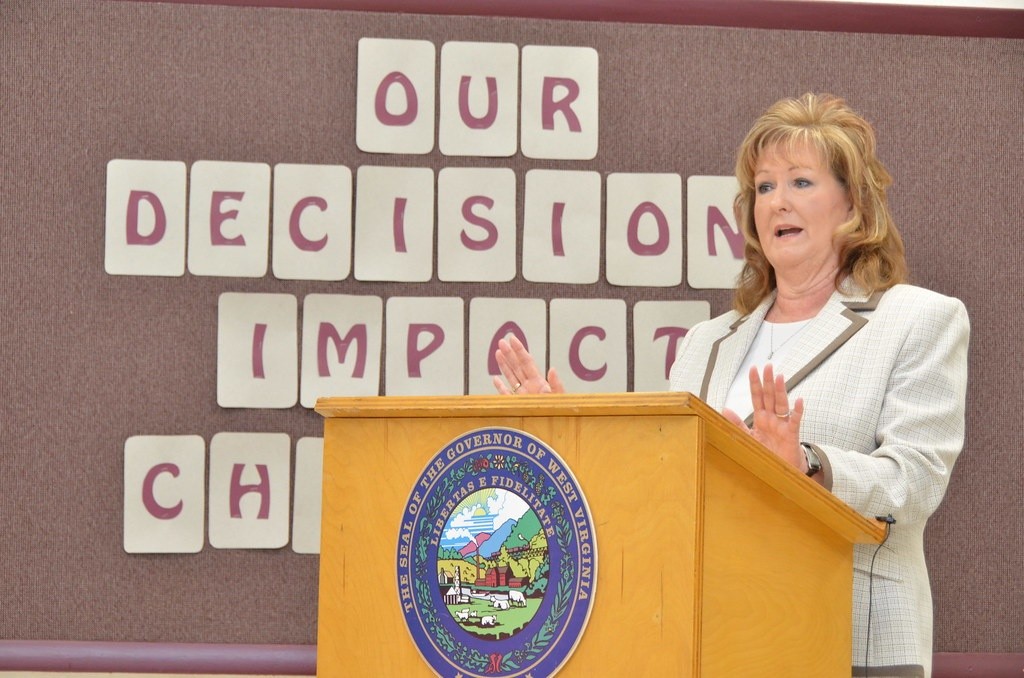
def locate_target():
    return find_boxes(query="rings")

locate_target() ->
[512,382,520,393]
[775,411,791,419]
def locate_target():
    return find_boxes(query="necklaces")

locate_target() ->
[767,294,816,361]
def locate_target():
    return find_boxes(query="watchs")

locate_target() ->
[799,442,821,478]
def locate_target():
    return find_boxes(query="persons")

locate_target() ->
[491,94,973,678]
